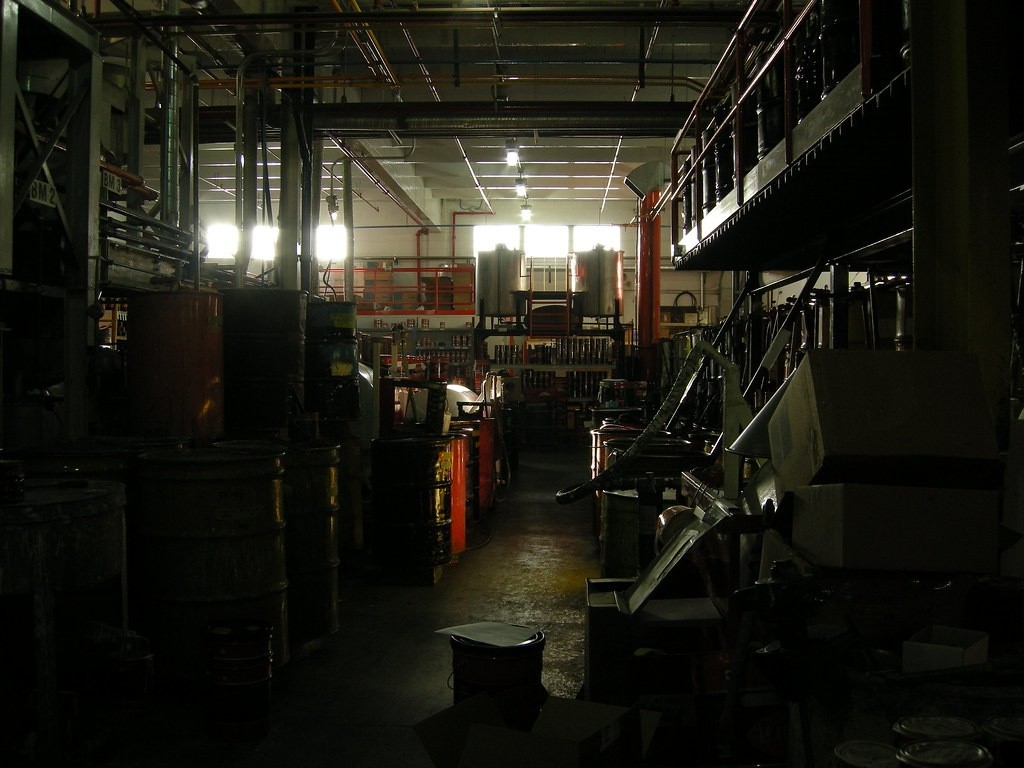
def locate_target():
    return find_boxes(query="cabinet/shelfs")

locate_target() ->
[358,329,614,402]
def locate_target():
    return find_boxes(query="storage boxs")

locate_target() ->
[412,334,1003,768]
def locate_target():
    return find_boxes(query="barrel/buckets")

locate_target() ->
[587,425,696,580]
[219,290,306,441]
[141,448,289,683]
[589,377,646,427]
[309,300,363,425]
[369,417,496,568]
[283,432,367,658]
[451,624,545,704]
[0,396,137,712]
[199,618,275,742]
[123,288,228,443]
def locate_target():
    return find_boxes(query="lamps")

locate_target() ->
[505,139,533,222]
